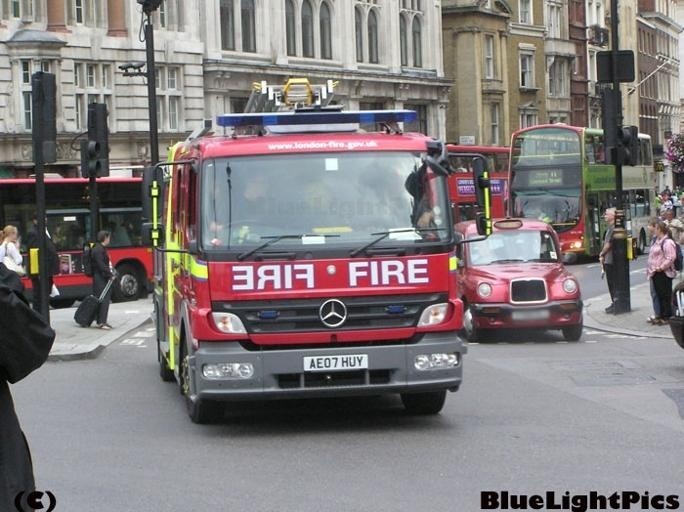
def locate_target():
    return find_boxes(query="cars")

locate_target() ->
[453,212,584,347]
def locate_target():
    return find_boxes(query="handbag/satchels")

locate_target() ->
[3,242,26,277]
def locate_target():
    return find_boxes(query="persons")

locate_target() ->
[598,207,618,313]
[210,156,434,233]
[452,154,494,174]
[473,237,497,265]
[646,186,684,326]
[1,211,119,512]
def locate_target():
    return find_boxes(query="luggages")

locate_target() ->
[74,277,115,328]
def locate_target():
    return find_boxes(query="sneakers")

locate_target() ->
[95,322,114,330]
[607,306,623,313]
[605,302,622,311]
[646,314,672,326]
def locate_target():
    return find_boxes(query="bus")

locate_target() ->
[0,174,152,306]
[443,123,660,262]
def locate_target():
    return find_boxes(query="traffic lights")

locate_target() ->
[86,141,100,175]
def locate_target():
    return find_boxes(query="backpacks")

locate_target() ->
[661,237,683,272]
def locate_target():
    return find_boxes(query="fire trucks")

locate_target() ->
[136,76,496,424]
